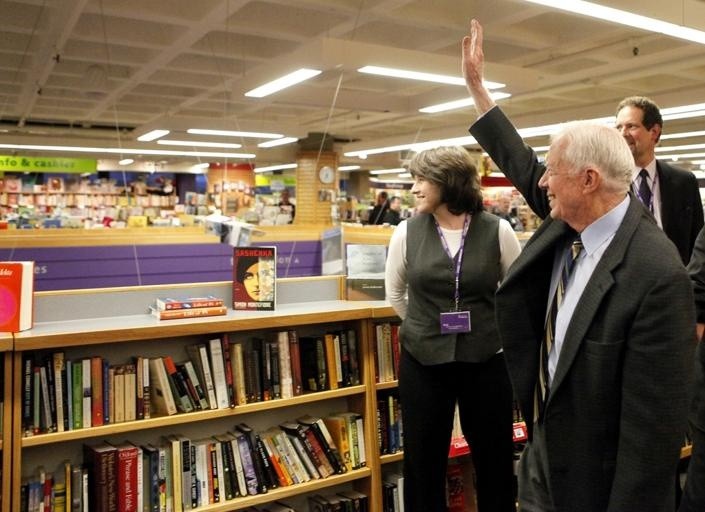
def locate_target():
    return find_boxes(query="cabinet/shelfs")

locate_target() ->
[1,173,180,224]
[0,297,431,507]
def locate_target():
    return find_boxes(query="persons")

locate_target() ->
[367,192,402,225]
[680,226,704,512]
[0,176,194,229]
[490,196,517,229]
[386,146,523,510]
[461,17,696,512]
[614,97,704,266]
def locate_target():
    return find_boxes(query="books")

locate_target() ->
[338,221,394,301]
[20,322,406,512]
[148,245,277,319]
[0,261,35,333]
[204,179,292,244]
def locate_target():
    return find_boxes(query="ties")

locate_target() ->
[636,169,654,213]
[532,236,583,422]
